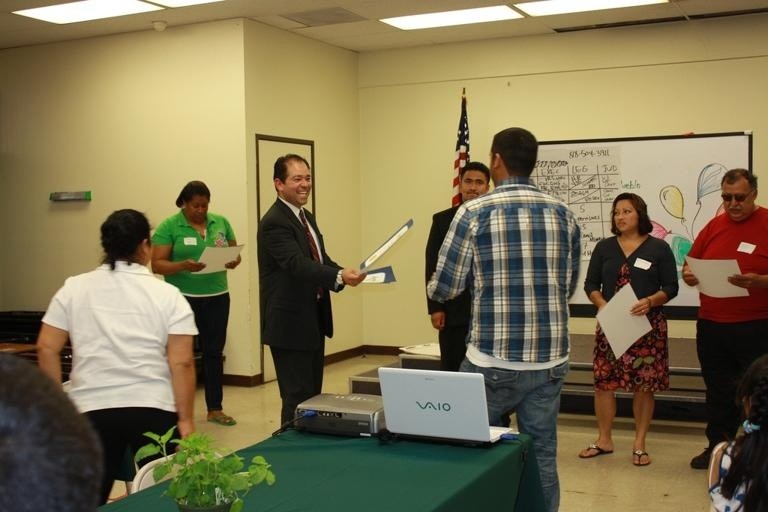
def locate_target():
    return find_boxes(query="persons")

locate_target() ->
[682,169,768,468]
[708,354,768,512]
[150,180,241,425]
[426,162,510,428]
[427,128,581,512]
[579,193,679,465]
[36,209,200,507]
[0,351,106,512]
[258,154,367,427]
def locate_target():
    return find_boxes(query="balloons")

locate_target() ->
[697,163,728,199]
[659,185,684,218]
[649,221,666,239]
[671,236,691,266]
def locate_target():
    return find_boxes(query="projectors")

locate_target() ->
[294,393,386,440]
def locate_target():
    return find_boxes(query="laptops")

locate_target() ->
[378,367,513,449]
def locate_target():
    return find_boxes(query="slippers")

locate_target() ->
[207,414,237,427]
[632,448,651,467]
[578,440,614,458]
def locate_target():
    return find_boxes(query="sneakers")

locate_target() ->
[690,448,713,470]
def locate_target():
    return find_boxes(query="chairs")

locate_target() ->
[130,447,228,493]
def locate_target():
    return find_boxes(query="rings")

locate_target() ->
[642,309,645,314]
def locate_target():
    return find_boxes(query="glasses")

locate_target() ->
[720,190,754,202]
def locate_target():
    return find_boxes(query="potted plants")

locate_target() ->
[130,425,280,512]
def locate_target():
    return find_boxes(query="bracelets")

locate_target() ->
[646,296,651,310]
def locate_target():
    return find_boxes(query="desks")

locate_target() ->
[0,337,43,360]
[91,402,537,512]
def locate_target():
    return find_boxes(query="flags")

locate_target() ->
[452,97,470,208]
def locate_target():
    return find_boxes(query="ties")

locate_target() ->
[299,209,325,298]
